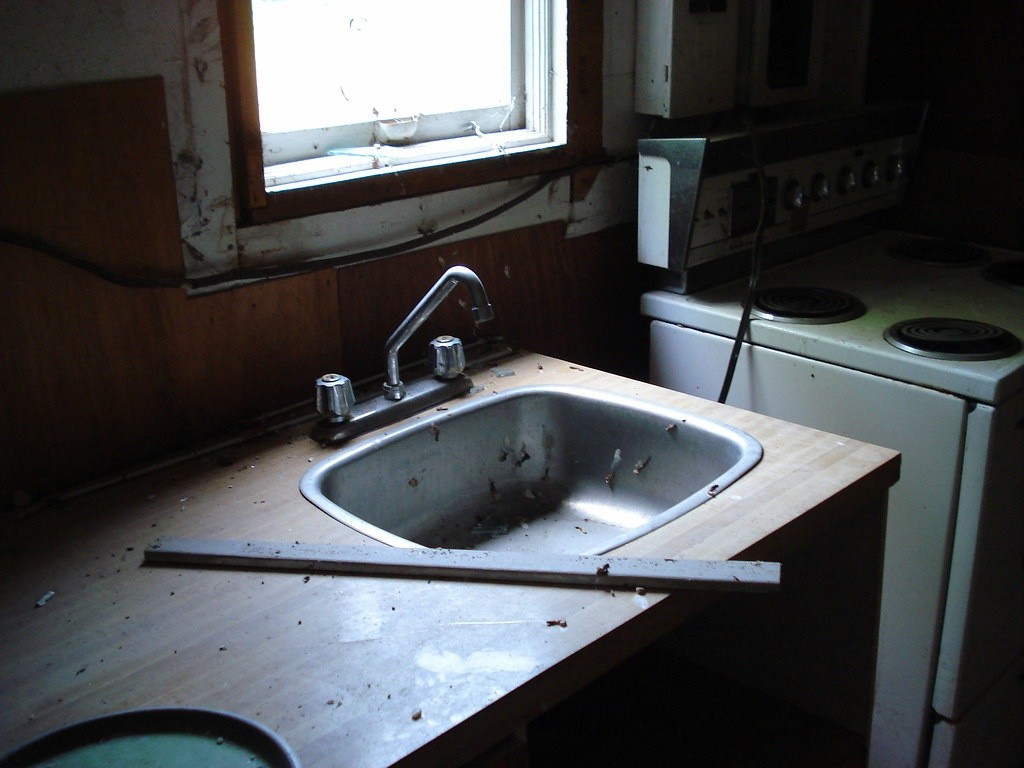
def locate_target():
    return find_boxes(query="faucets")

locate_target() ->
[382,264,494,401]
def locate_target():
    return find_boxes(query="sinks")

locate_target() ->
[298,375,765,570]
